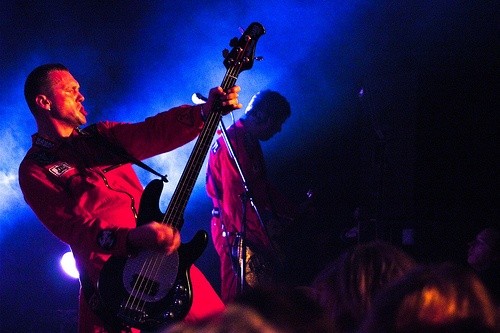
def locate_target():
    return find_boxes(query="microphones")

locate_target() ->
[191,93,208,105]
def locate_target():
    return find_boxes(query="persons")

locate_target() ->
[206,92,291,333]
[248,228,500,333]
[19,63,244,333]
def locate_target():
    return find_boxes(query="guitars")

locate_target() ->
[231,177,350,288]
[93,21,266,332]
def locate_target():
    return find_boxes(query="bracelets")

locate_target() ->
[200,104,207,121]
